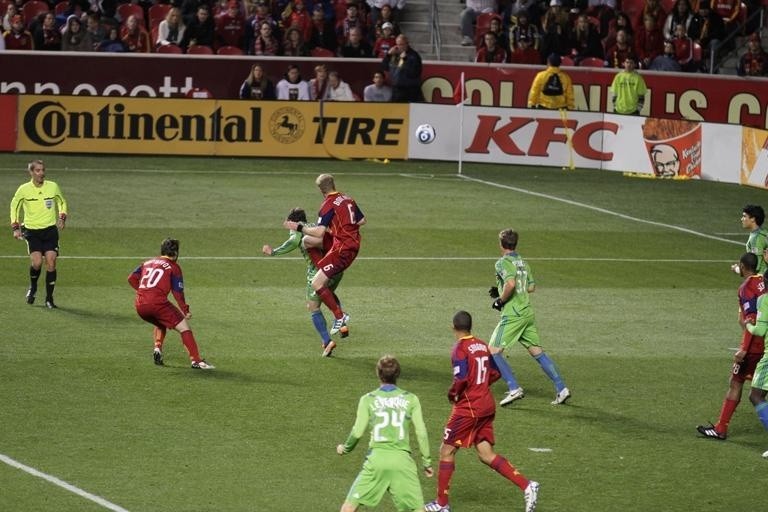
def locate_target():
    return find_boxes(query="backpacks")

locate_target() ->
[544,72,564,96]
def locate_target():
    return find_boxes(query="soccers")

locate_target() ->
[415,124,437,144]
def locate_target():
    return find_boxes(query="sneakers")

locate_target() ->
[23,286,37,305]
[549,386,572,406]
[522,479,541,512]
[191,357,216,370]
[423,498,451,512]
[338,314,350,339]
[45,297,58,310]
[319,340,336,358]
[499,386,525,407]
[693,421,729,441]
[329,312,351,337]
[153,347,164,365]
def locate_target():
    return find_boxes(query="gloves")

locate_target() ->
[487,286,500,299]
[491,298,504,311]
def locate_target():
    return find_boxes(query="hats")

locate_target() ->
[381,21,394,32]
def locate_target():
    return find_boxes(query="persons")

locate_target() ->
[63,16,90,51]
[247,0,274,55]
[3,15,34,50]
[99,29,128,52]
[545,3,571,33]
[739,34,768,78]
[308,6,339,55]
[374,24,397,56]
[527,55,574,109]
[337,356,434,512]
[382,34,423,103]
[710,0,741,30]
[663,1,698,43]
[589,1,615,24]
[671,23,692,72]
[458,1,498,45]
[609,55,646,114]
[156,9,185,45]
[36,13,61,51]
[652,42,678,71]
[2,1,162,18]
[312,66,332,102]
[745,270,767,457]
[573,15,599,57]
[695,252,767,440]
[481,19,506,46]
[284,174,366,334]
[241,66,275,99]
[9,159,67,308]
[487,230,571,407]
[255,22,278,55]
[375,5,402,37]
[329,71,355,102]
[261,207,350,357]
[639,15,661,57]
[639,0,666,27]
[185,7,211,50]
[507,34,538,64]
[476,33,508,65]
[607,29,638,64]
[123,15,148,52]
[364,72,392,101]
[218,3,248,48]
[341,3,364,46]
[425,311,540,511]
[339,25,372,56]
[290,1,311,37]
[277,64,310,100]
[284,26,307,56]
[511,13,540,49]
[731,204,767,275]
[612,14,635,43]
[127,238,215,370]
